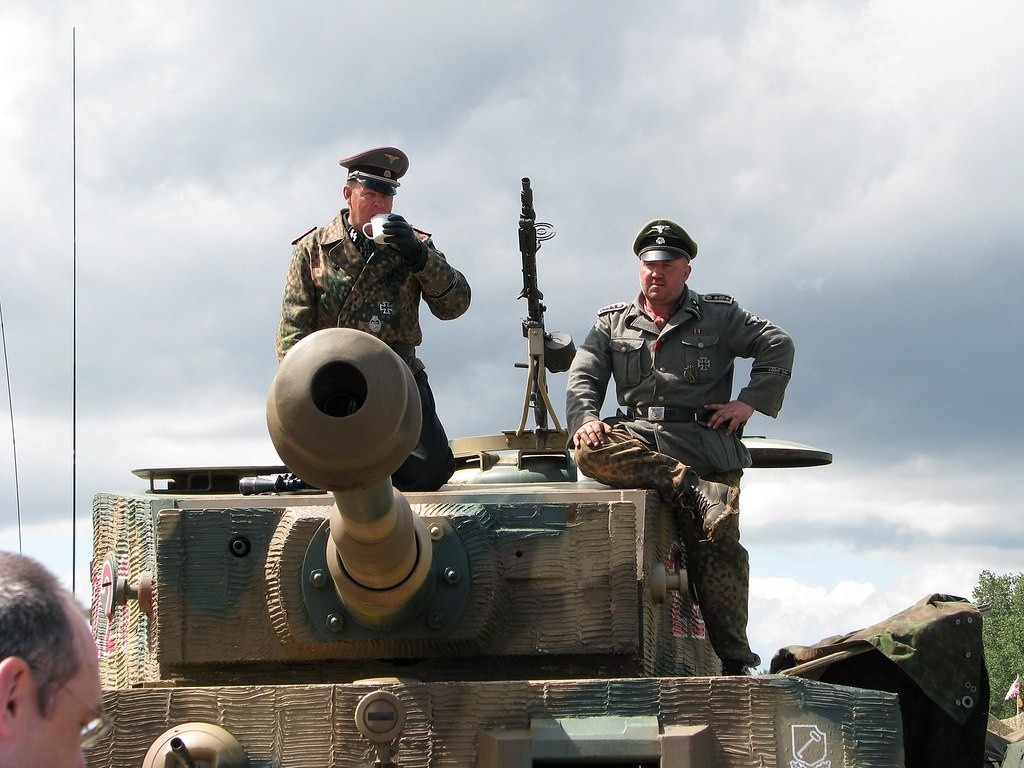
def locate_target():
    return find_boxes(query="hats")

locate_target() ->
[633,219,698,264]
[338,146,409,195]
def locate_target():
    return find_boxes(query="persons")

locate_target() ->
[567,219,794,675]
[0,551,102,768]
[278,147,472,493]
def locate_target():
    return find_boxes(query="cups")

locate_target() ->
[362,213,396,244]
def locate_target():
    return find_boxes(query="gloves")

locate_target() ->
[382,214,421,268]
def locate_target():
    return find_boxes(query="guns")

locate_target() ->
[512,175,552,438]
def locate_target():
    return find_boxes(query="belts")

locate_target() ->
[626,406,715,423]
[388,343,415,358]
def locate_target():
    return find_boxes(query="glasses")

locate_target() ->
[28,663,113,749]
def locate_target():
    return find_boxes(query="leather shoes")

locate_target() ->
[721,663,752,675]
[678,471,740,545]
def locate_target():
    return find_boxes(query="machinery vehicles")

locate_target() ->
[77,177,904,768]
